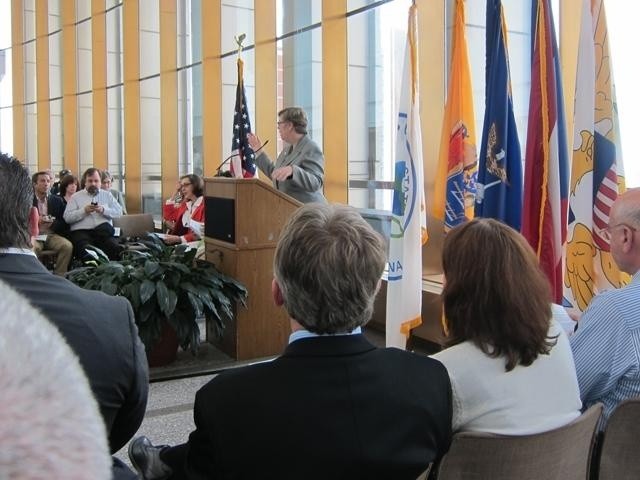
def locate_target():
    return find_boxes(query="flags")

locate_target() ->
[474,1,522,233]
[386,2,427,352]
[230,60,260,179]
[564,1,632,310]
[432,0,479,236]
[522,0,571,305]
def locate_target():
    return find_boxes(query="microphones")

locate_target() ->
[214,139,269,177]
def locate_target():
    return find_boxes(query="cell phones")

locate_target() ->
[91,202,97,205]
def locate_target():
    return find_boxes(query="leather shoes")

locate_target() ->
[129,437,175,480]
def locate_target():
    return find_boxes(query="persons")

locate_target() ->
[127,202,453,480]
[564,186,640,434]
[0,153,150,455]
[429,218,584,436]
[29,167,127,279]
[248,107,326,205]
[0,279,113,479]
[161,174,206,246]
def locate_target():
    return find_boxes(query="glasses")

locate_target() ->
[598,224,636,240]
[180,182,192,187]
[276,120,290,125]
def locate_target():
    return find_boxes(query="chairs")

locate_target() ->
[598,399,640,480]
[436,402,604,480]
[113,213,155,238]
[40,250,59,273]
[416,461,433,480]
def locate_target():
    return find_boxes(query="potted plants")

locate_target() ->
[65,233,248,365]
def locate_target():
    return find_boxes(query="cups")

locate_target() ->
[90,196,98,210]
